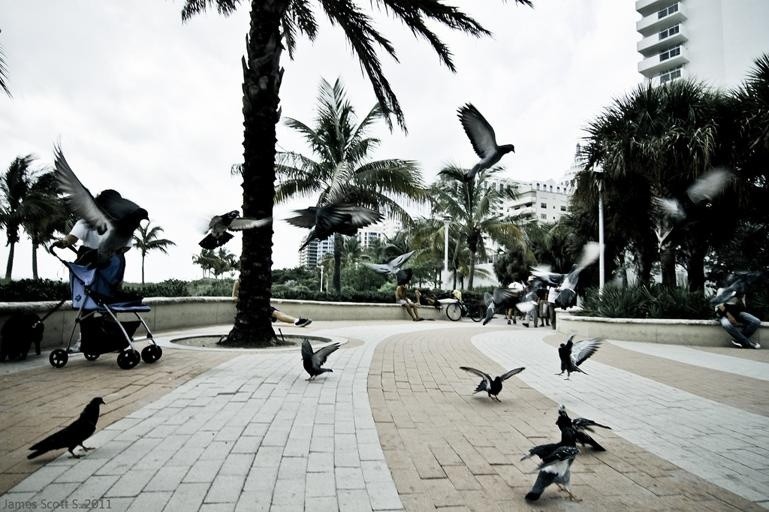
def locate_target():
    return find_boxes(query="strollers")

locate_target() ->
[45,234,164,371]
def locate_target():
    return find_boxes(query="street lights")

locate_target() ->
[438,212,453,294]
[318,259,324,294]
[591,158,611,312]
[354,261,359,271]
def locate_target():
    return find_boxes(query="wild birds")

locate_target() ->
[25,397,107,460]
[285,202,385,252]
[520,404,611,504]
[361,249,416,286]
[300,337,341,382]
[557,335,604,380]
[481,241,604,326]
[50,141,150,272]
[457,102,516,183]
[459,365,525,404]
[199,209,273,250]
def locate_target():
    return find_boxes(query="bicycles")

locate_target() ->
[445,289,484,323]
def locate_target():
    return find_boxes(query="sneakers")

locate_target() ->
[730,337,761,350]
[411,315,426,322]
[503,314,556,330]
[294,316,313,328]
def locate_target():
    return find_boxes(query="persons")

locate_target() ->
[504,278,556,329]
[395,281,424,321]
[62,189,131,354]
[232,273,312,327]
[715,281,763,349]
